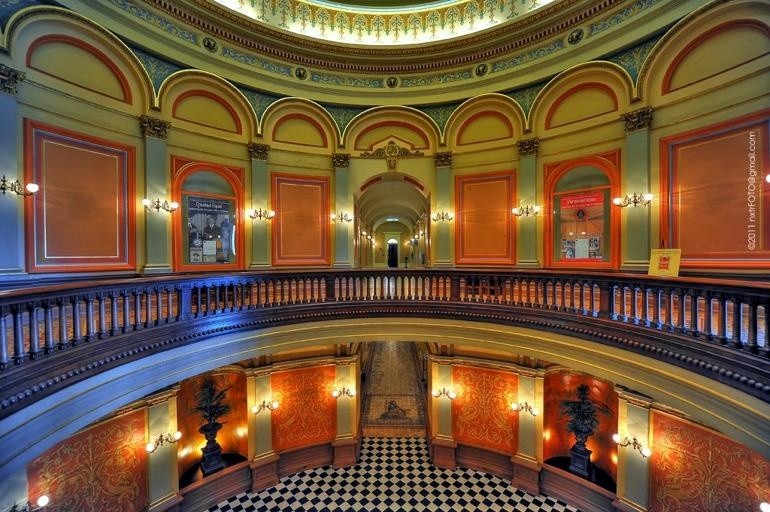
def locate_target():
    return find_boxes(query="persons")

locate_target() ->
[202,214,223,238]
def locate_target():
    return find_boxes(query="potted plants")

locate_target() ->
[561,384,600,455]
[192,379,234,454]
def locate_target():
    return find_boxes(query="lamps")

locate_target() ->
[431,385,459,400]
[509,399,540,417]
[431,189,654,226]
[612,432,651,458]
[7,496,49,511]
[250,399,278,415]
[330,386,356,399]
[145,429,182,454]
